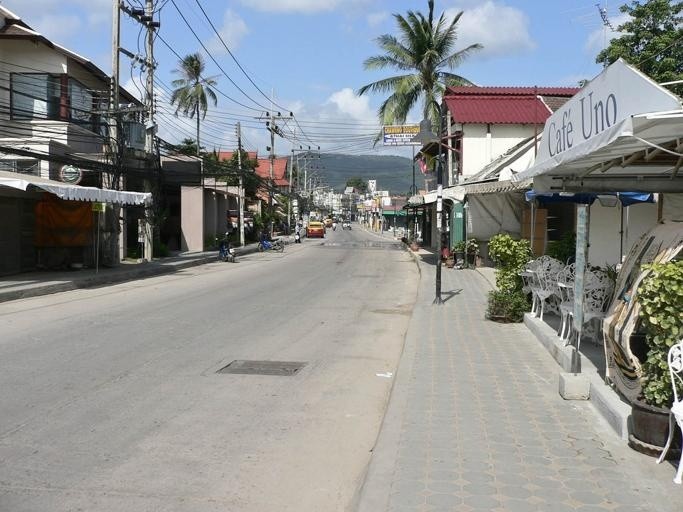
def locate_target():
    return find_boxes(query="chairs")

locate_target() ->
[656,343,683,484]
[518,255,615,346]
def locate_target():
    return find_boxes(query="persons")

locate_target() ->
[295,223,301,243]
[281,221,288,235]
[333,217,350,224]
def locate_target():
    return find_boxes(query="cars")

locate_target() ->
[342,217,351,231]
[306,222,324,238]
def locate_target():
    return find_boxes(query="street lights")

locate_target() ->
[410,99,443,305]
[408,185,419,241]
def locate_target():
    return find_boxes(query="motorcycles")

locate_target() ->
[258,231,284,253]
[332,217,337,230]
[215,232,236,263]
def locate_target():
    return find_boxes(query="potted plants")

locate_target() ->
[629,260,683,460]
[452,238,480,265]
[446,255,455,267]
